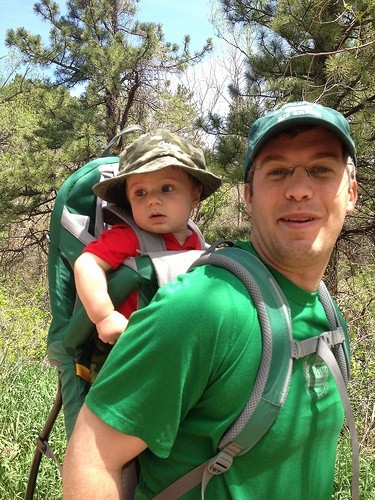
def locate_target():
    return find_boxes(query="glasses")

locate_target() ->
[255,157,353,180]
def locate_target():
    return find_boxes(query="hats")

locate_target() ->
[243,101,357,180]
[92,128,222,205]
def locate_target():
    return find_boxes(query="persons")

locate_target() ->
[46,101,360,500]
[71,127,224,389]
